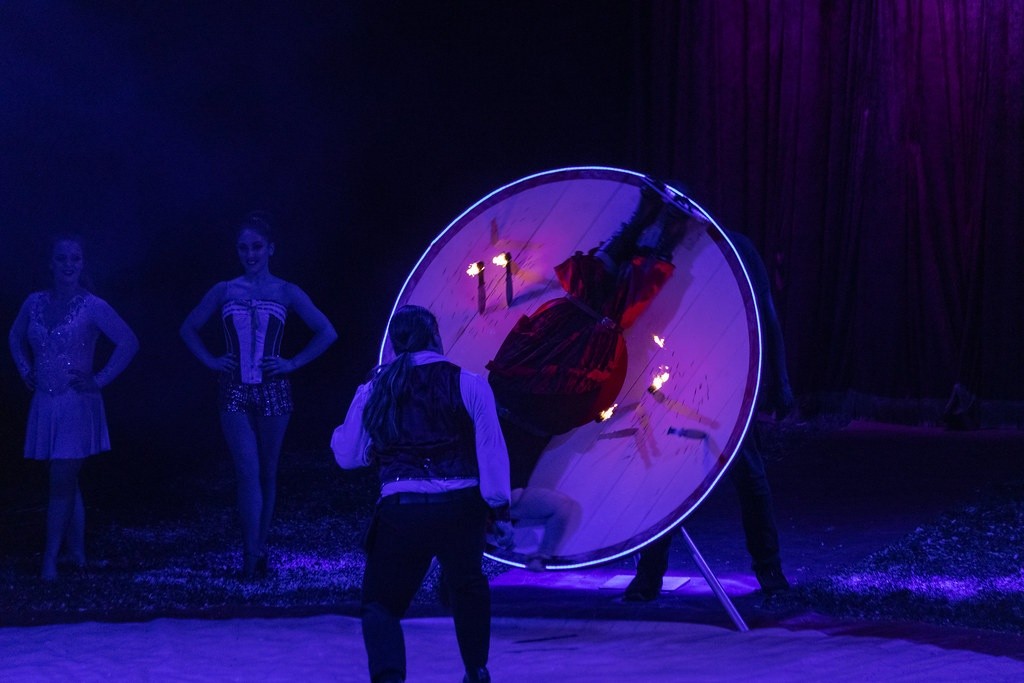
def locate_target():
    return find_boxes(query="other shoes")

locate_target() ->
[463,667,492,683]
[246,551,268,580]
[38,577,58,596]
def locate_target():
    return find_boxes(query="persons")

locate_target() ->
[602,172,791,601]
[8,234,140,576]
[179,220,337,580]
[328,305,511,683]
[482,174,694,573]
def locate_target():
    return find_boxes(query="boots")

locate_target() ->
[597,176,664,266]
[644,197,690,252]
